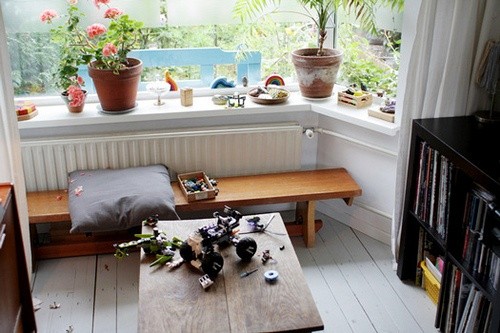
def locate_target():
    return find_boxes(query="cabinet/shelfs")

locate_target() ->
[0,182,38,333]
[398,115,500,333]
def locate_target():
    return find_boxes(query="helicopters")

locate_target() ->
[112,227,183,267]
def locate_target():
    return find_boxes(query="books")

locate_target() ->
[414,143,500,333]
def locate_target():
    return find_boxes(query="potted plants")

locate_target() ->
[233,0,407,98]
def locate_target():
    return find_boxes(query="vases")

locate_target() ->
[61,90,88,113]
[88,57,142,110]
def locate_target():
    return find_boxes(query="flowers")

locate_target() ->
[39,0,144,107]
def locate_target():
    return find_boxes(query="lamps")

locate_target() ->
[474,39,500,124]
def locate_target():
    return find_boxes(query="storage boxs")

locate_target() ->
[339,90,372,108]
[421,260,440,304]
[180,87,192,106]
[177,170,215,202]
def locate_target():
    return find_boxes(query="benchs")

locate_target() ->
[26,167,362,260]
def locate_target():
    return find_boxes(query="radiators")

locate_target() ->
[20,121,302,235]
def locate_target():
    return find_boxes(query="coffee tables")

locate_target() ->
[135,213,324,333]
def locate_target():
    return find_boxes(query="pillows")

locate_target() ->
[68,164,180,234]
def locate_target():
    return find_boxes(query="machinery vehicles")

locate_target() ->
[179,205,257,278]
